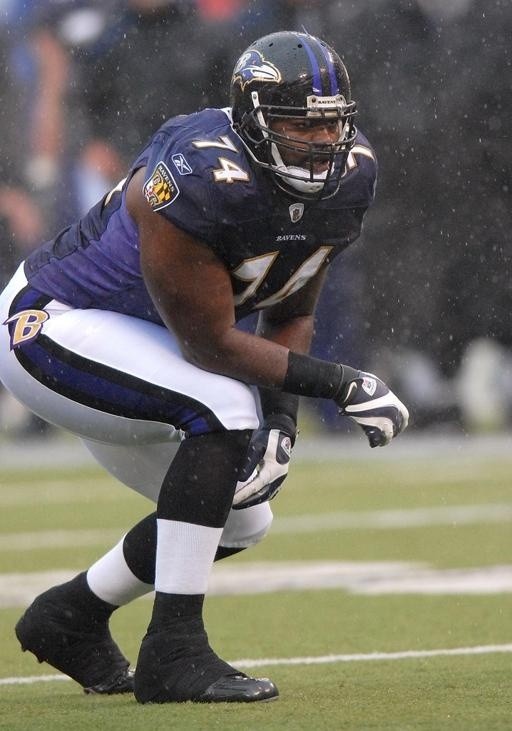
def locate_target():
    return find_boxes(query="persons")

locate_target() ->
[0,24,409,708]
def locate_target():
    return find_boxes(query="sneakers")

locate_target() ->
[200,674,279,704]
[83,666,138,694]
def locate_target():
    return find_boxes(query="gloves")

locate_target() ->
[344,368,410,447]
[230,426,294,510]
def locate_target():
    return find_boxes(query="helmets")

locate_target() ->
[228,29,359,204]
